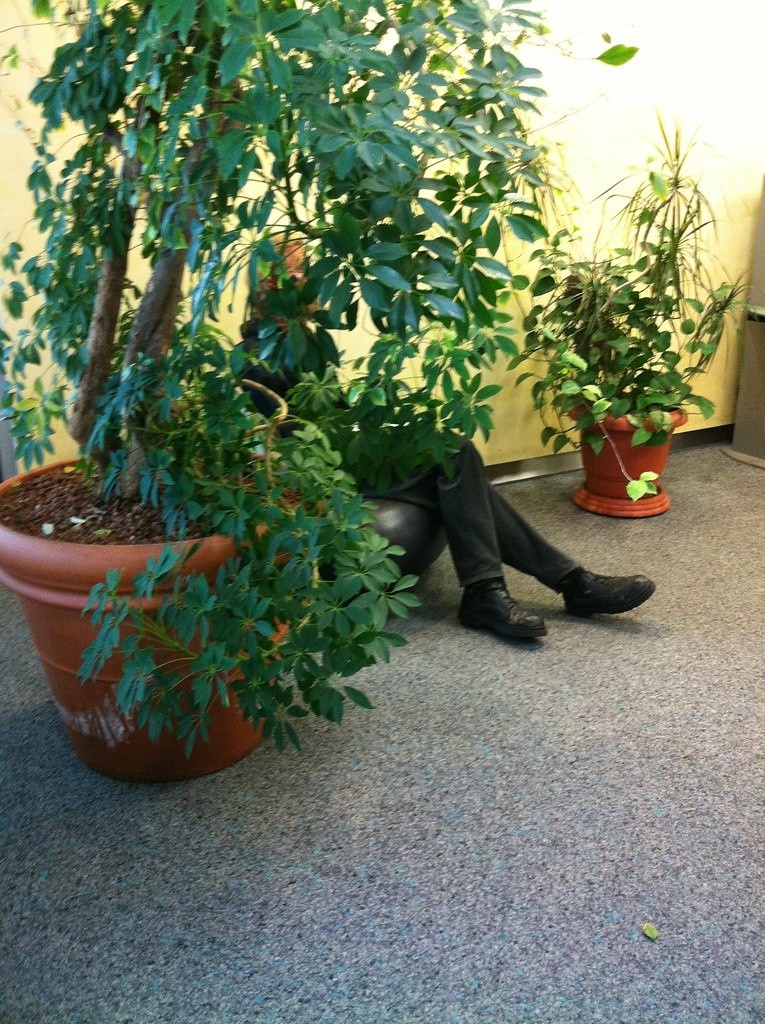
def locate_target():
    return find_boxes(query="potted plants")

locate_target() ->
[526,114,756,518]
[0,0,566,785]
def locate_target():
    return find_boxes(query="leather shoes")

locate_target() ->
[460,595,547,640]
[563,572,655,617]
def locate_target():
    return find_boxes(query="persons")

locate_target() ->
[225,228,662,639]
